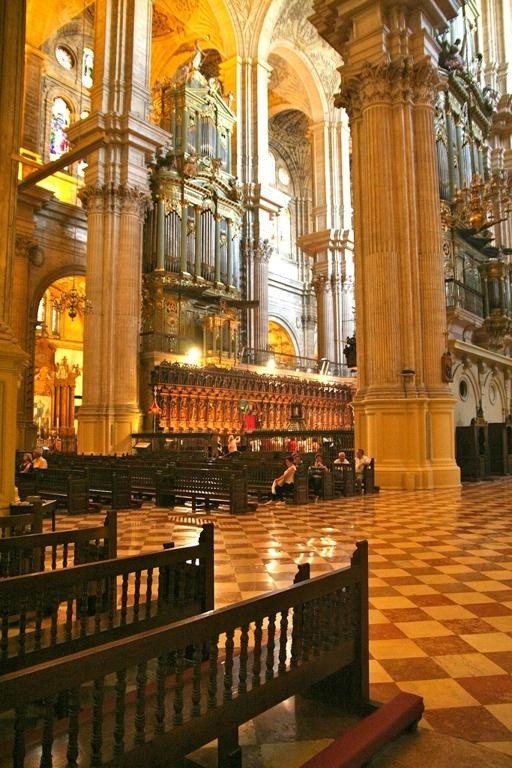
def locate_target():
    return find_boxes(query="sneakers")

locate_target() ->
[266,500,285,505]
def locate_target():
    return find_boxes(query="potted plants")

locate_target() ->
[344,332,355,368]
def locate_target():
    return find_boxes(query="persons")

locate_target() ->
[181,38,234,109]
[17,452,32,473]
[216,434,372,506]
[31,448,48,478]
[155,141,239,187]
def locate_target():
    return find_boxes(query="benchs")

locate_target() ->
[15,452,375,510]
[0,493,425,768]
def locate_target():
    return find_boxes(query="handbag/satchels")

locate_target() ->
[278,479,285,487]
